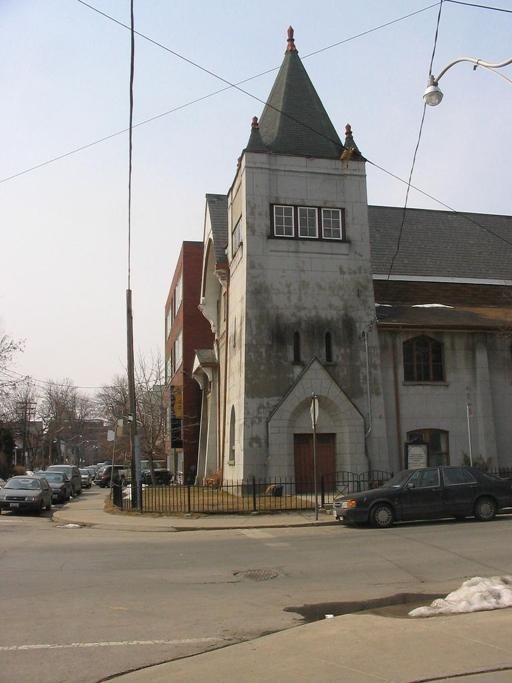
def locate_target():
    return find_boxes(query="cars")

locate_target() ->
[0,460,173,513]
[333,466,512,528]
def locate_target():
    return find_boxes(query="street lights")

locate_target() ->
[422,57,512,106]
